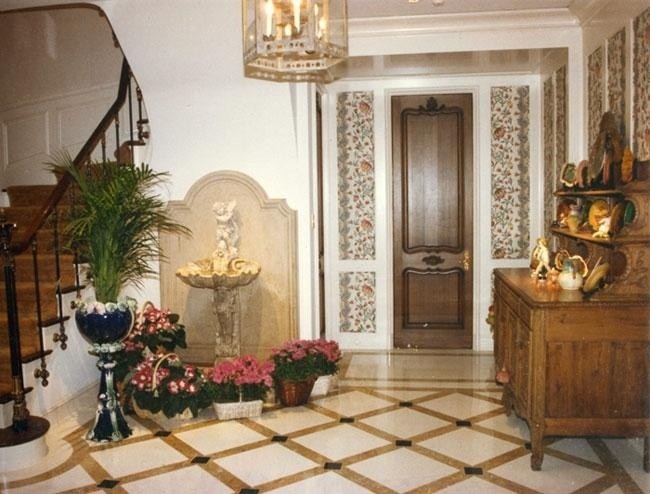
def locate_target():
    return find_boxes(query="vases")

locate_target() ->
[213,400,262,421]
[308,375,332,396]
[131,393,200,426]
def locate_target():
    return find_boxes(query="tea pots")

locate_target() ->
[557,260,582,290]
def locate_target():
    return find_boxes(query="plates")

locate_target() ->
[587,198,608,230]
[584,262,610,293]
[577,159,592,186]
[610,201,625,233]
[622,202,635,225]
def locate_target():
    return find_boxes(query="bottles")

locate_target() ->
[566,208,583,231]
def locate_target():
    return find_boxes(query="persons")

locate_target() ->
[533,236,552,277]
[211,199,239,259]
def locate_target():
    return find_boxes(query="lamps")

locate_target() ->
[238,0,357,84]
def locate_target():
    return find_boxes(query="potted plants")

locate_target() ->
[41,143,194,443]
[271,361,317,407]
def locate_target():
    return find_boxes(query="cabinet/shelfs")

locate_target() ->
[493,110,650,474]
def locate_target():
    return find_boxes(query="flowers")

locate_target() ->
[268,336,344,376]
[116,301,188,370]
[200,352,277,403]
[126,348,212,419]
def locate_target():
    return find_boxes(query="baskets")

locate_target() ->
[131,353,194,419]
[264,375,332,406]
[212,392,263,420]
